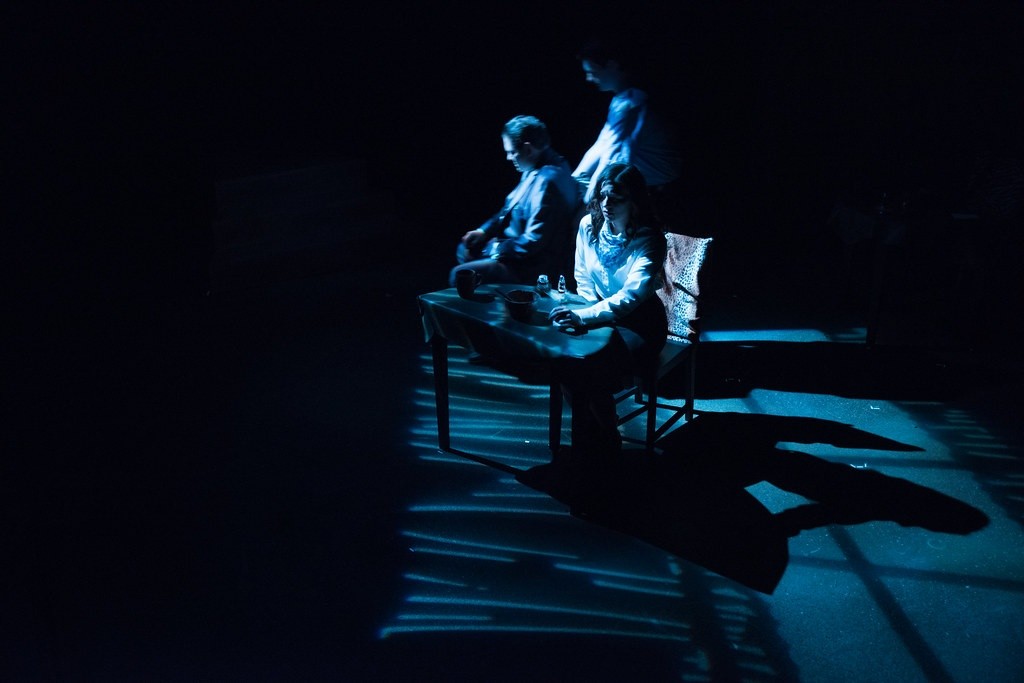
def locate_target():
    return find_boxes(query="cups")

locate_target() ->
[456,270,483,298]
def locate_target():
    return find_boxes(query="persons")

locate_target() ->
[450,44,667,365]
[548,164,669,516]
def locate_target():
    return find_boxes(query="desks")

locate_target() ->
[423,280,617,509]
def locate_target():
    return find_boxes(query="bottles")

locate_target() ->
[558,275,565,300]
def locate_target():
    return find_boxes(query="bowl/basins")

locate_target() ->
[506,290,543,319]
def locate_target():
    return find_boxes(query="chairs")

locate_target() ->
[545,231,717,496]
